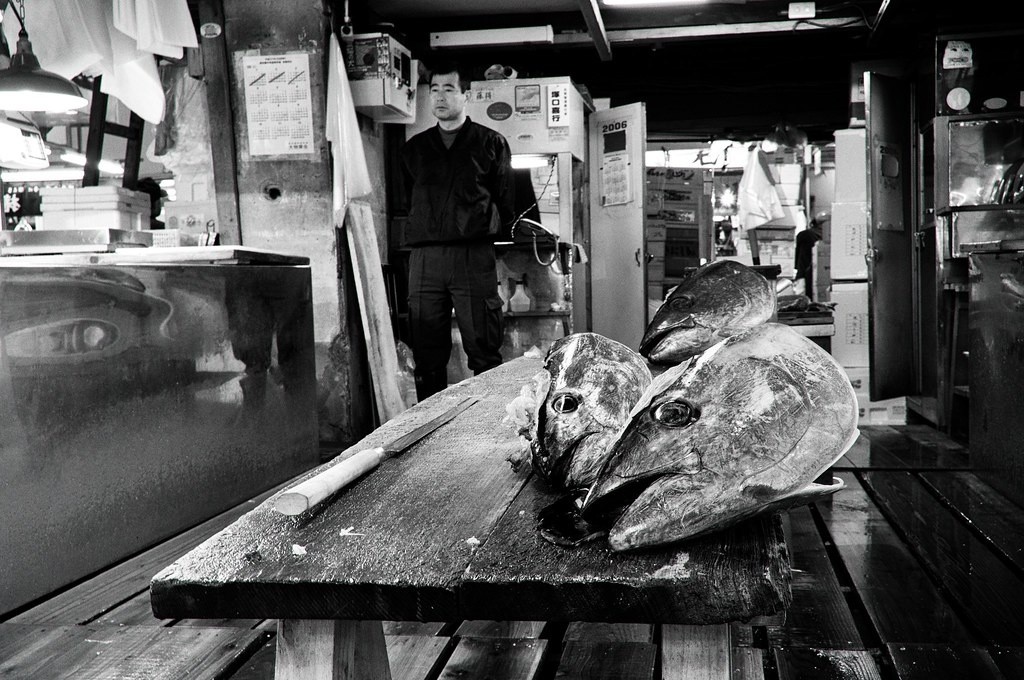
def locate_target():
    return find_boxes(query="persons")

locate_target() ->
[223,269,314,430]
[398,65,512,403]
[137,177,168,230]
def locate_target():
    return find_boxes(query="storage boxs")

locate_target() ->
[830,129,908,425]
[38,188,150,230]
[767,163,804,205]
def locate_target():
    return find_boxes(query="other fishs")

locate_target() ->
[639,260,778,368]
[528,326,656,489]
[581,323,861,552]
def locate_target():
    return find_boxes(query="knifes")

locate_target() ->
[279,395,481,516]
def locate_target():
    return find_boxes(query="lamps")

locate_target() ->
[0,0,89,110]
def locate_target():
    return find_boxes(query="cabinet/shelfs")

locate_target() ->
[396,239,572,337]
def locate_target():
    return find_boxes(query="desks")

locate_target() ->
[153,346,789,680]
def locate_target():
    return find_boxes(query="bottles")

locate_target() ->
[496,280,508,312]
[522,273,536,312]
[510,279,530,312]
